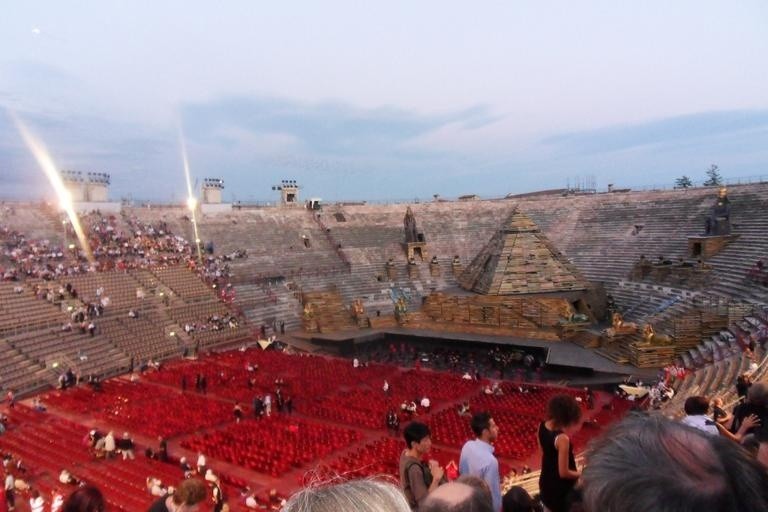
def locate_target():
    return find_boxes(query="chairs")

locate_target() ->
[1,265,253,403]
[0,346,652,512]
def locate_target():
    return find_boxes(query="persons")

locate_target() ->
[233,399,244,425]
[254,395,265,420]
[181,373,188,391]
[199,376,208,395]
[271,319,278,333]
[260,324,266,338]
[264,395,273,417]
[196,373,200,390]
[272,338,767,511]
[2,204,246,337]
[0,387,225,512]
[279,318,286,335]
[56,366,102,391]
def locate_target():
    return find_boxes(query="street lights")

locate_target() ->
[202,177,225,203]
[187,196,197,239]
[280,179,299,206]
[59,196,74,242]
[85,172,111,203]
[58,170,85,202]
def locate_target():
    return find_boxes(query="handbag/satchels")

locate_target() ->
[403,463,449,506]
